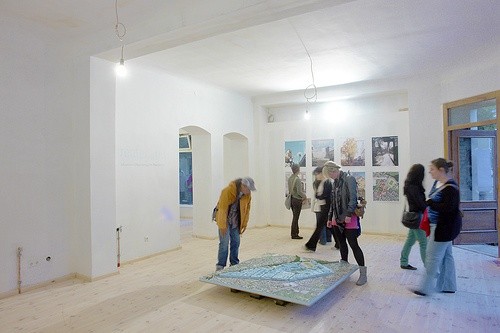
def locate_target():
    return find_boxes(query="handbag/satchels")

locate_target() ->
[283,193,292,211]
[401,210,422,230]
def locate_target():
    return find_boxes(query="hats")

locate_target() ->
[241,177,256,191]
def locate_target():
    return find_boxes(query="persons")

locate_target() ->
[322,161,367,286]
[288,164,306,239]
[303,165,346,251]
[216,177,257,271]
[400,164,428,270]
[406,158,463,296]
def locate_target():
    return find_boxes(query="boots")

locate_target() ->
[356,266,367,285]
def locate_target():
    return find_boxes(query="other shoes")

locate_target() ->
[400,264,417,270]
[330,245,339,250]
[301,245,314,251]
[291,236,303,239]
